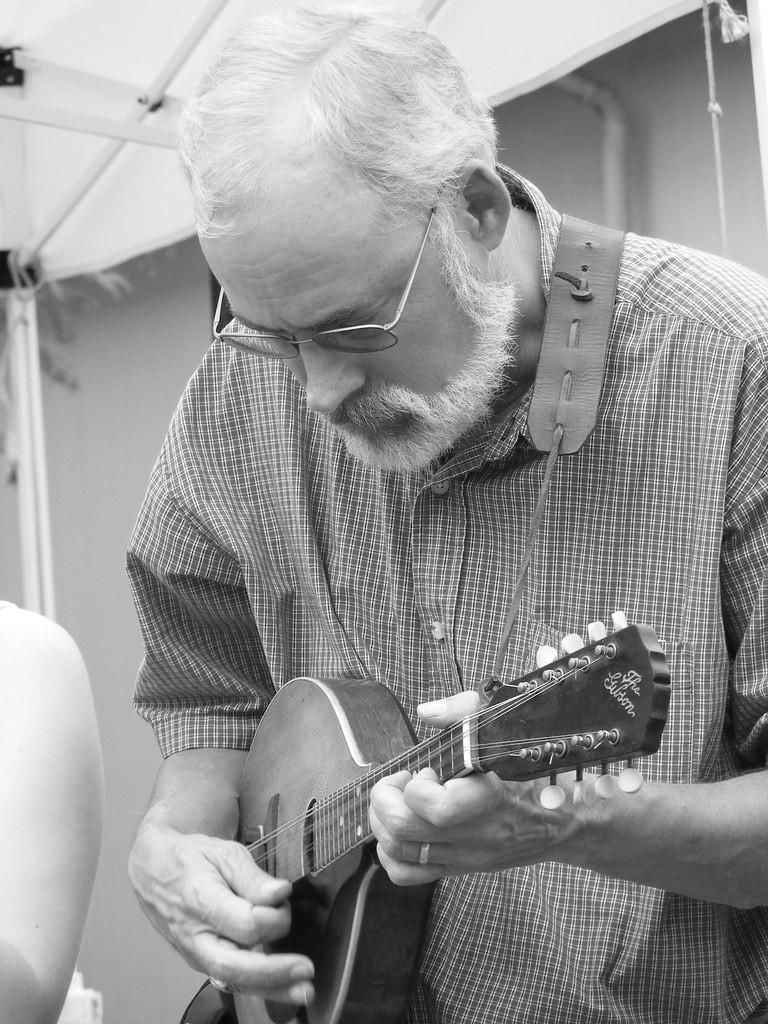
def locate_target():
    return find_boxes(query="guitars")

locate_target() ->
[228,609,672,1023]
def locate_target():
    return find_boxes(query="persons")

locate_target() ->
[125,10,768,1024]
[0,602,106,1024]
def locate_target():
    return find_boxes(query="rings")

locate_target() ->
[419,842,430,865]
[209,976,232,993]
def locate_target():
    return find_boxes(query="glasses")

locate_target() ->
[211,158,502,360]
